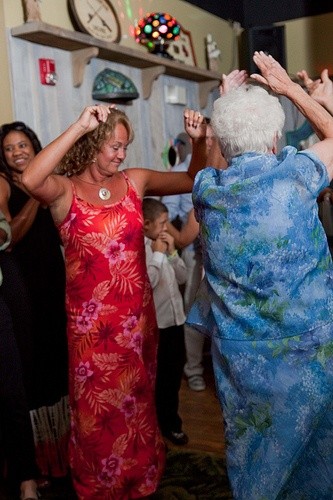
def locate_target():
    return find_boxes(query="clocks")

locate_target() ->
[67,0,122,44]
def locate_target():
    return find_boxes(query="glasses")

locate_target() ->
[0,122,27,131]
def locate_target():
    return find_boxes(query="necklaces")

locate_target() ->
[75,175,110,201]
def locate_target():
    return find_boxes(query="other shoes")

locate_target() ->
[188,375,206,391]
[163,432,198,455]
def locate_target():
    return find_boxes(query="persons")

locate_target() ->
[0,121,71,500]
[141,117,229,445]
[190,50,333,500]
[298,67,333,115]
[22,103,209,500]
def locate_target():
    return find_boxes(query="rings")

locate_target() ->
[196,112,201,118]
[271,61,275,64]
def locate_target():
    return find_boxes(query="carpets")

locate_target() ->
[37,451,235,500]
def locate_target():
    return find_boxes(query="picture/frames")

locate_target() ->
[167,29,197,66]
